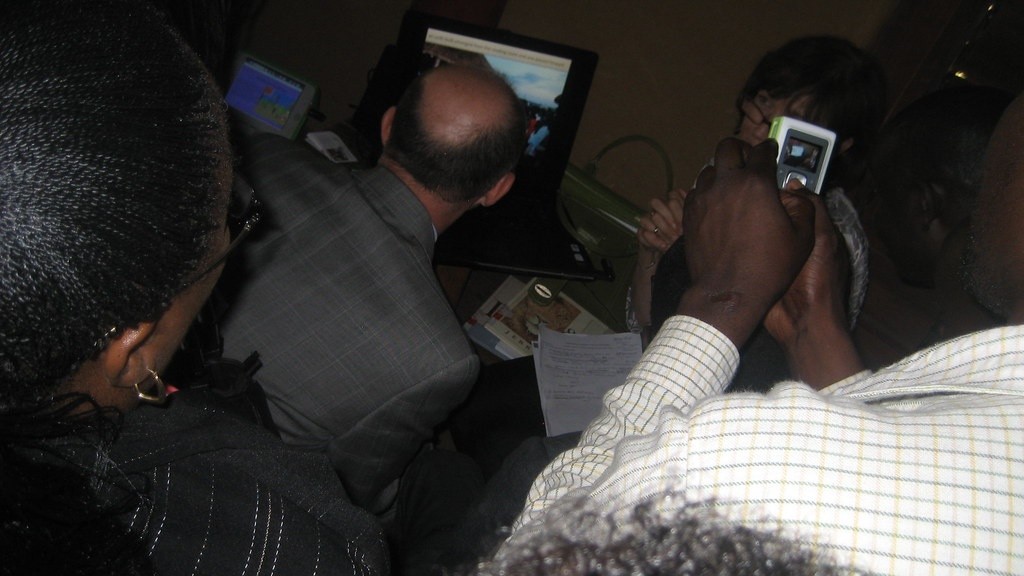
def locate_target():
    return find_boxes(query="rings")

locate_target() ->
[654,228,660,233]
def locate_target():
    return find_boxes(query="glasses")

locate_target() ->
[737,91,771,131]
[71,156,261,372]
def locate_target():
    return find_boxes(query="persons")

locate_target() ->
[391,87,1024,575]
[0,0,394,576]
[635,32,898,392]
[163,63,529,509]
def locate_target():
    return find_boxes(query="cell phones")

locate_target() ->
[767,115,837,198]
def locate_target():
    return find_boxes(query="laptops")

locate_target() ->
[375,10,599,283]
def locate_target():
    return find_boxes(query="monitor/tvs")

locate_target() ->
[214,51,322,141]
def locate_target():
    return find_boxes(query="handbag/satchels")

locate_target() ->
[559,136,673,334]
